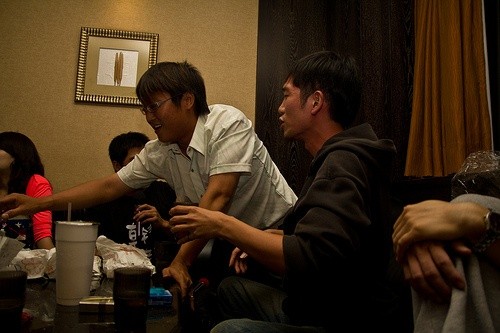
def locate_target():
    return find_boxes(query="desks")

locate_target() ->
[24,276,178,333]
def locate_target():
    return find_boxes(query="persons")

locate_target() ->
[79,131,178,269]
[393,193,500,333]
[0,132,54,250]
[0,60,300,310]
[167,50,403,333]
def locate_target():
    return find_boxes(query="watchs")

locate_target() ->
[473,207,500,254]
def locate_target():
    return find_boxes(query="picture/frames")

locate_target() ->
[74,27,159,106]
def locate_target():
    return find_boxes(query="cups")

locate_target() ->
[135,205,148,223]
[0,271,27,315]
[172,202,199,242]
[55,221,99,306]
[112,267,152,325]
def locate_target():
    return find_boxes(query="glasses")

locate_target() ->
[139,97,171,116]
